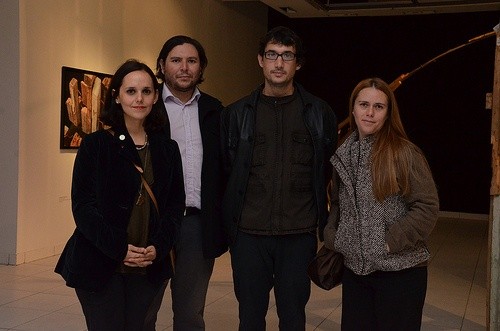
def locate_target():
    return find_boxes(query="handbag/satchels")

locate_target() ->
[309,214,343,290]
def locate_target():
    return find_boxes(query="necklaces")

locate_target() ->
[136,134,148,150]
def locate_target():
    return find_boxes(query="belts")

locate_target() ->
[183,207,200,216]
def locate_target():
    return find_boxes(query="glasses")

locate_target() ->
[266,51,294,60]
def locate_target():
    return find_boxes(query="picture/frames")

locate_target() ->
[60,66,114,149]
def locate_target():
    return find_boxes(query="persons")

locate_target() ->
[144,35,229,331]
[324,79,440,331]
[54,58,186,331]
[220,27,338,331]
